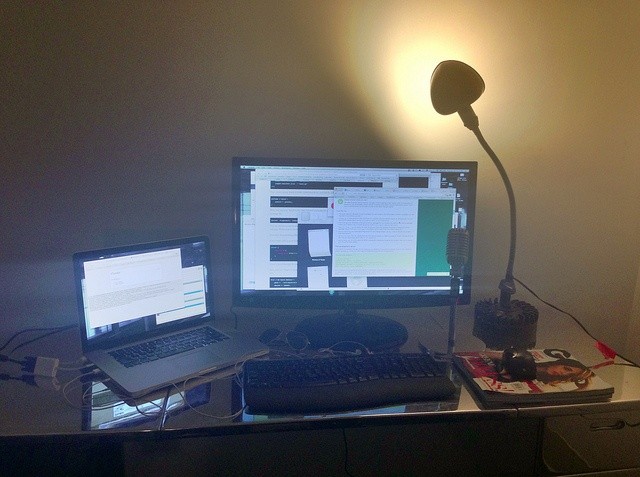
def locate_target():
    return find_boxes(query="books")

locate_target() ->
[450,363,612,409]
[453,349,614,402]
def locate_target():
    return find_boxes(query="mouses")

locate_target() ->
[501,344,537,380]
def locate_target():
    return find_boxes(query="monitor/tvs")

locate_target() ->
[229,153,480,353]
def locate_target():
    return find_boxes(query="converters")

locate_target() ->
[31,354,61,379]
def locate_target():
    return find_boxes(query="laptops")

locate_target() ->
[80,353,268,430]
[74,235,270,397]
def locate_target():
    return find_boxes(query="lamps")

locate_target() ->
[432,62,540,346]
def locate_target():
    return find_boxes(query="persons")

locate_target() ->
[458,350,595,393]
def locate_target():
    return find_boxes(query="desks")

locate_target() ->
[2,321,639,475]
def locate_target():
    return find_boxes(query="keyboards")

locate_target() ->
[240,351,457,412]
